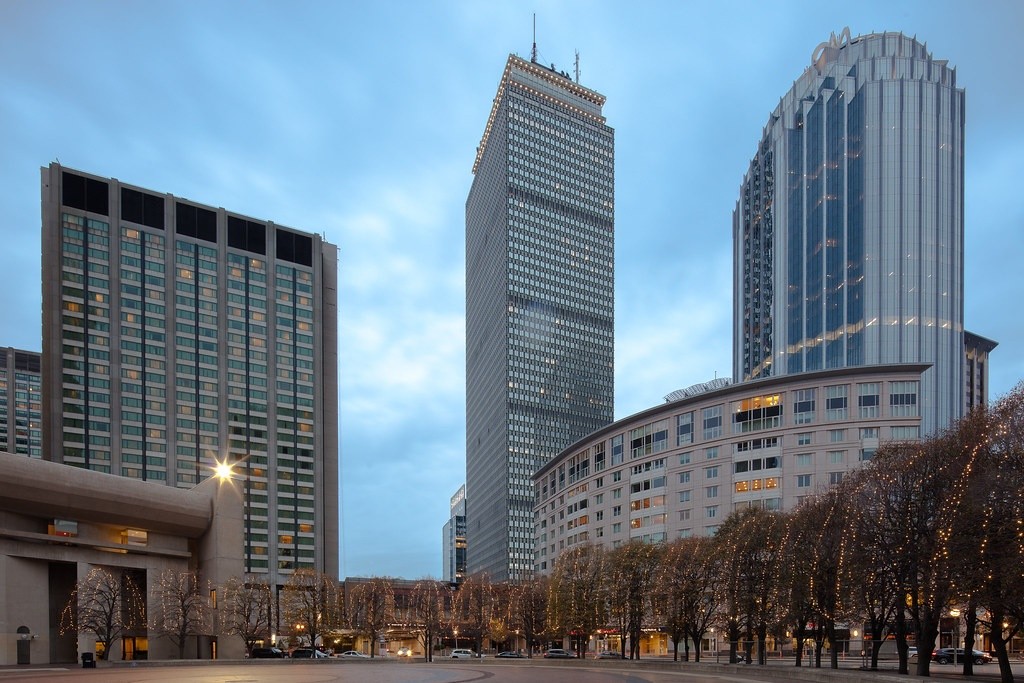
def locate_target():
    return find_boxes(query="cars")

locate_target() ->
[396,646,412,656]
[253,648,283,658]
[343,651,368,657]
[449,648,486,659]
[594,651,630,659]
[936,647,992,665]
[495,651,528,658]
[292,649,328,658]
[908,647,918,658]
[543,648,575,658]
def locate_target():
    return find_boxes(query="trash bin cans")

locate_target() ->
[81,651,93,668]
[681,655,686,661]
